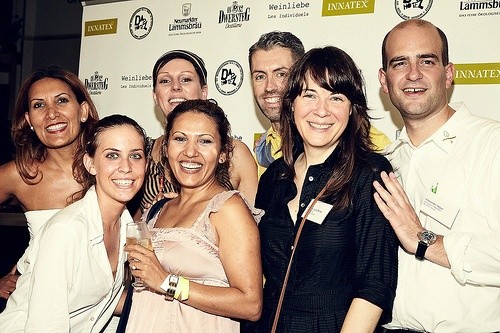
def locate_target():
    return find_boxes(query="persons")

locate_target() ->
[1,112,153,333]
[250,45,400,333]
[0,66,101,311]
[246,29,394,183]
[122,98,265,332]
[133,48,259,220]
[377,17,499,333]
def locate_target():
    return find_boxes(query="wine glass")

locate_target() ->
[126,221,154,287]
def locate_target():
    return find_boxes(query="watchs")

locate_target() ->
[415,230,438,262]
[164,273,180,301]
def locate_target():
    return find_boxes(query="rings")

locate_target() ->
[132,262,137,270]
